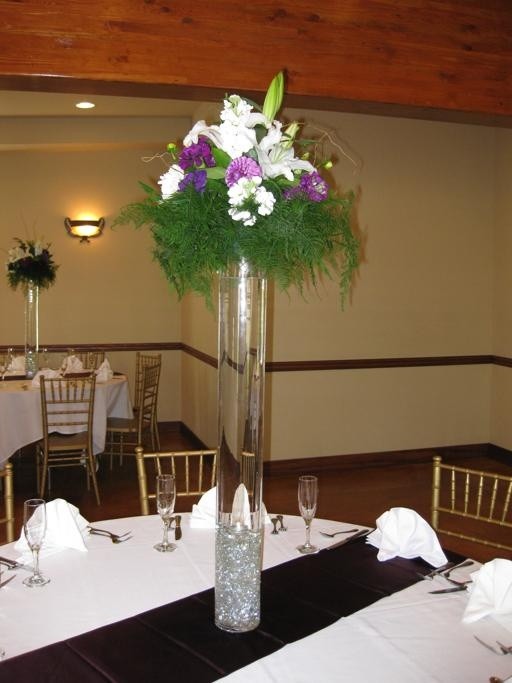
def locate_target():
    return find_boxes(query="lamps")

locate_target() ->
[64,216,104,245]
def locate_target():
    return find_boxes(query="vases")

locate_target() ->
[214,275,268,631]
[23,282,39,371]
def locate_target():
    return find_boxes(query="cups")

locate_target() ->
[39,349,48,370]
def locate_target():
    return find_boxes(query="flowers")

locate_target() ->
[3,232,60,298]
[114,71,362,495]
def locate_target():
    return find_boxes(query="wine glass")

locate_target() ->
[297,475,318,555]
[153,475,178,553]
[0,348,13,382]
[23,499,50,588]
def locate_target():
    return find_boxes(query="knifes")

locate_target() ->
[0,556,34,572]
[325,529,369,550]
[428,587,467,594]
[174,515,183,541]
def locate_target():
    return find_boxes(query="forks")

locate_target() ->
[277,515,286,532]
[0,561,17,569]
[270,518,278,534]
[431,561,474,578]
[87,526,132,538]
[89,532,133,543]
[320,528,359,537]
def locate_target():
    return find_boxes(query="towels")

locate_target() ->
[62,355,81,373]
[32,368,65,389]
[367,502,447,570]
[463,556,512,629]
[18,498,87,562]
[189,483,277,537]
[93,360,113,384]
[5,357,26,371]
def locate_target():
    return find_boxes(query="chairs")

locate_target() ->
[1,464,17,544]
[67,349,104,371]
[37,374,102,505]
[133,351,161,451]
[134,445,223,514]
[431,456,512,563]
[104,364,160,470]
